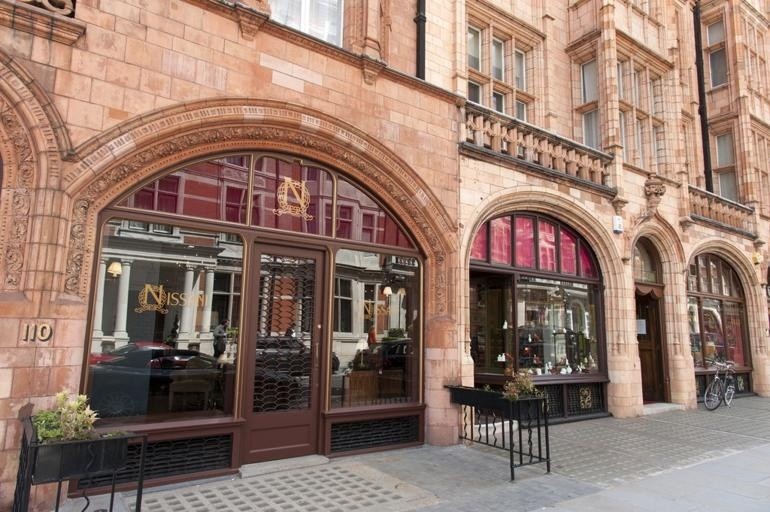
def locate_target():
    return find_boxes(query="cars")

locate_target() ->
[517,324,576,345]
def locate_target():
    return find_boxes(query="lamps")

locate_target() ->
[637,318,646,335]
[613,215,624,233]
[357,339,369,367]
[383,287,406,297]
[107,262,122,277]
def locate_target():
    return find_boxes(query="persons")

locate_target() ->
[367,326,377,349]
[285,323,297,337]
[212,318,232,360]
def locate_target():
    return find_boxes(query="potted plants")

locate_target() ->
[451,369,541,419]
[25,391,135,485]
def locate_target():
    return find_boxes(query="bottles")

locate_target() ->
[544,363,549,374]
[497,353,505,361]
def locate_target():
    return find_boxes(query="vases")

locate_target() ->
[504,368,514,377]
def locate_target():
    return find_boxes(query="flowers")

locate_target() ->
[504,353,514,369]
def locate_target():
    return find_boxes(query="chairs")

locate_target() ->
[168,358,219,412]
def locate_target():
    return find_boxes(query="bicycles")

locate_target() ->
[703,357,736,411]
